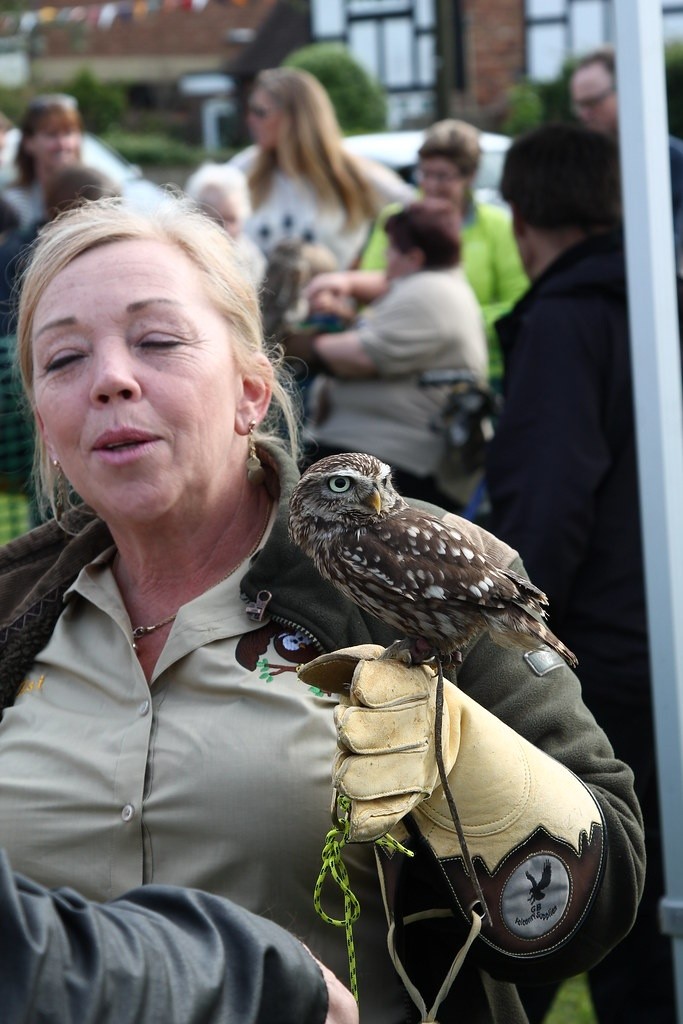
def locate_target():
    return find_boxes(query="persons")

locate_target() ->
[485,124,679,1024]
[0,52,683,513]
[0,182,645,1024]
[0,842,361,1024]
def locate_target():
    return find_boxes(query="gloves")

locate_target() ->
[299,644,610,959]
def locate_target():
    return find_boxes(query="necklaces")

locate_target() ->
[131,610,175,656]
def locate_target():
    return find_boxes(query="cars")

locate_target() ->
[344,127,519,218]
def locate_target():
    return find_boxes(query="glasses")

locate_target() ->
[246,102,283,118]
[572,90,616,108]
